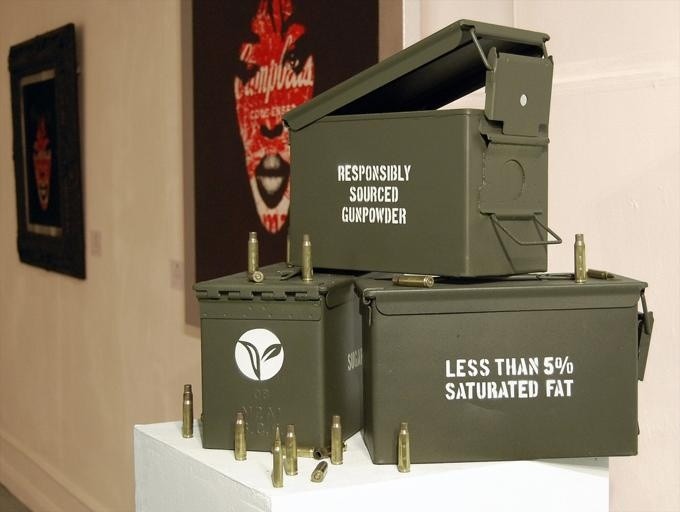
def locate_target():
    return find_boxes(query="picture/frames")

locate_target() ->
[191,0,379,283]
[8,22,85,280]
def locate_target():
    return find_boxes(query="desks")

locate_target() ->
[135,420,610,512]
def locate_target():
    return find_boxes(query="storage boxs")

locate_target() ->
[193,263,362,457]
[354,273,654,464]
[281,19,552,279]
